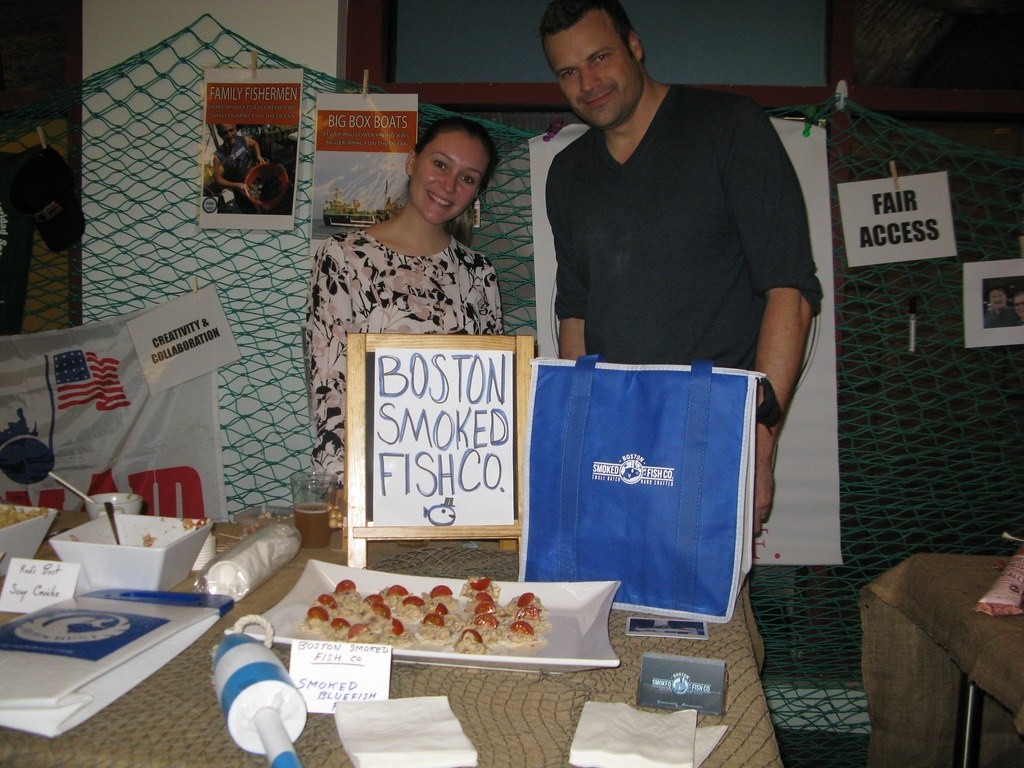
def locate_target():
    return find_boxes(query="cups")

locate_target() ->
[191,524,303,603]
[291,472,338,549]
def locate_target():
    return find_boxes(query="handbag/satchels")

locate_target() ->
[518,350,781,623]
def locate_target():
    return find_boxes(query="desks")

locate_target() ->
[856,552,1024,768]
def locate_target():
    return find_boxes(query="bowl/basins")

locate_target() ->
[233,505,297,538]
[48,513,214,591]
[86,492,144,521]
[0,504,59,575]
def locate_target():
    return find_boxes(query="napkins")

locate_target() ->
[568,701,728,768]
[331,695,478,768]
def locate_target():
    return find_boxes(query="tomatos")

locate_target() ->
[307,577,541,643]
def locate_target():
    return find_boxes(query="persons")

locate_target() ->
[209,123,267,215]
[537,0,824,667]
[301,115,503,512]
[983,285,1024,328]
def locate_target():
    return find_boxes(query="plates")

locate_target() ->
[224,558,622,675]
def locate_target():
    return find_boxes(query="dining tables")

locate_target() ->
[0,502,785,768]
